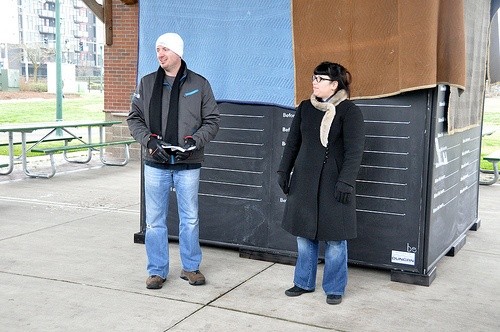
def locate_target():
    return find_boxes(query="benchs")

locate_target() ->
[483,151,500,185]
[0,136,137,178]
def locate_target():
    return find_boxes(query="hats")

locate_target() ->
[155,32,184,59]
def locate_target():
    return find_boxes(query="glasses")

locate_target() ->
[312,75,331,83]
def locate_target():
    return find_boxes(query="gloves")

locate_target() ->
[277,171,289,194]
[175,136,196,164]
[334,181,353,205]
[147,134,172,163]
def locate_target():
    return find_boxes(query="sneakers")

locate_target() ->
[285,285,315,296]
[146,275,166,289]
[327,294,342,304]
[180,269,205,285]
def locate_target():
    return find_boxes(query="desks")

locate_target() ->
[0,121,122,178]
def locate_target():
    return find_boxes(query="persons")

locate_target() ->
[276,61,364,304]
[125,33,221,289]
